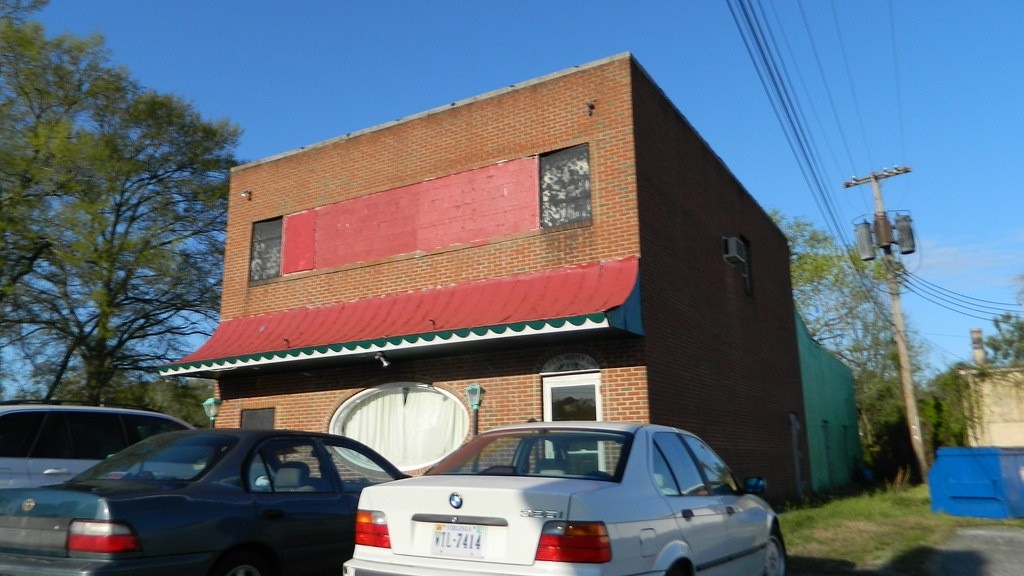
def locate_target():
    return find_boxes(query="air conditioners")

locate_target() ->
[723,236,745,264]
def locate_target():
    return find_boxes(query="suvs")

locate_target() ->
[0,402,198,488]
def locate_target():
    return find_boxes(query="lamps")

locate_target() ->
[241,189,251,199]
[376,351,390,367]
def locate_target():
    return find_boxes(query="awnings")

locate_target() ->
[156,254,644,379]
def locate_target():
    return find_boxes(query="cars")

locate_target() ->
[342,420,787,576]
[0,428,413,576]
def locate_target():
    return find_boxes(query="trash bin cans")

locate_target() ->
[928,446,1024,519]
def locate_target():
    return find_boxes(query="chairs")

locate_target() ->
[535,457,570,476]
[275,467,317,494]
